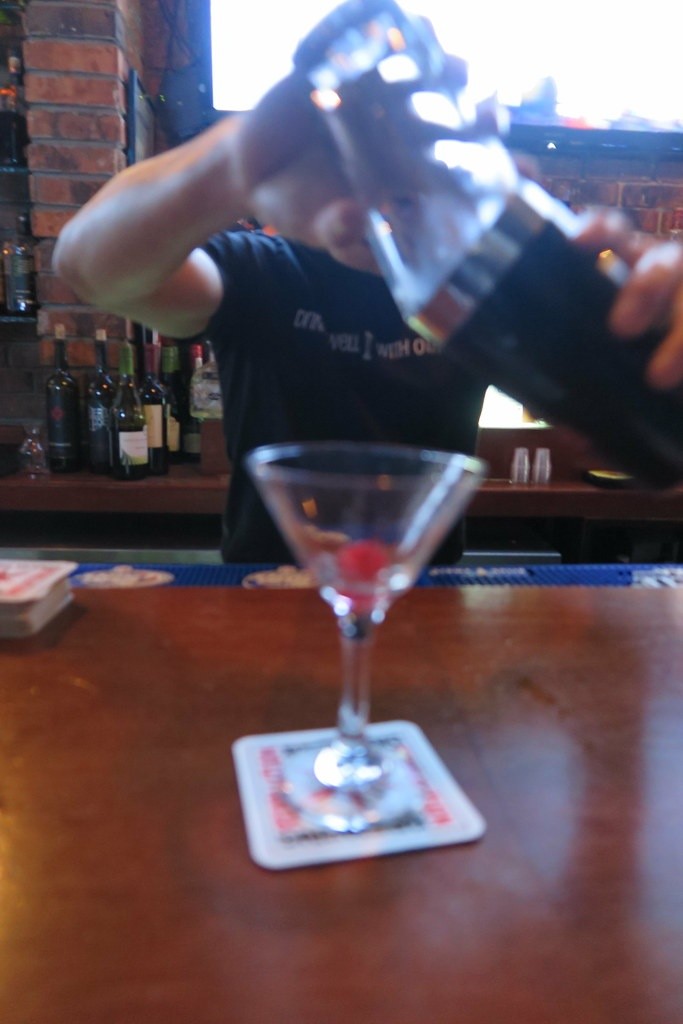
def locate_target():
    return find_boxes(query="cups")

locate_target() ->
[532,447,552,483]
[292,0,519,320]
[405,179,683,484]
[509,447,530,483]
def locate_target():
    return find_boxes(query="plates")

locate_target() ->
[242,568,318,590]
[79,569,175,589]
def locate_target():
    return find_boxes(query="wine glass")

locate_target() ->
[244,441,491,833]
[17,422,48,477]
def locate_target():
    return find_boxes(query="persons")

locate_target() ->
[48,13,683,565]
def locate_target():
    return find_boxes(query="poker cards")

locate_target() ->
[229,717,492,873]
[0,557,77,641]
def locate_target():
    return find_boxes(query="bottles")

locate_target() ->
[44,323,80,472]
[3,214,36,315]
[137,344,168,475]
[159,346,182,464]
[84,329,119,474]
[0,55,28,168]
[182,343,202,463]
[111,339,149,480]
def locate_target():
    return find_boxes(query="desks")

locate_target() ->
[0,587,683,1024]
